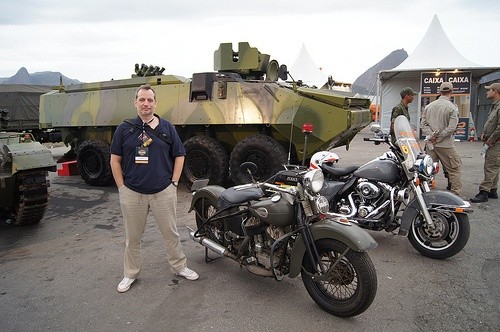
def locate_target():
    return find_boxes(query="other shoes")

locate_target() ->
[470,191,488,203]
[179,268,199,280]
[488,189,498,199]
[117,278,135,292]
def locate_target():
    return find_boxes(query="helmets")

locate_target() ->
[310,151,339,171]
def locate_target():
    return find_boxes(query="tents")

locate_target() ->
[376,13,500,140]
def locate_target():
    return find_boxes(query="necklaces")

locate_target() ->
[147,118,154,123]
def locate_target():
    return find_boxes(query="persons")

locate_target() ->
[110,85,199,293]
[470,83,500,203]
[389,87,418,144]
[420,82,462,197]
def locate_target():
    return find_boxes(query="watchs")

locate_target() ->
[172,181,179,186]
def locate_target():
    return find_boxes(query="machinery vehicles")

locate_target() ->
[39,41,374,193]
[0,109,57,228]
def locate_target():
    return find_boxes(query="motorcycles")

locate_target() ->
[274,114,475,259]
[185,161,378,317]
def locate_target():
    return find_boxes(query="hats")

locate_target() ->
[484,83,500,89]
[440,82,453,91]
[25,130,31,133]
[400,87,418,99]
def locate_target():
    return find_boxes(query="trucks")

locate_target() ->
[0,84,66,143]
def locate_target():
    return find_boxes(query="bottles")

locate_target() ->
[426,132,434,150]
[480,143,489,158]
[24,134,33,143]
